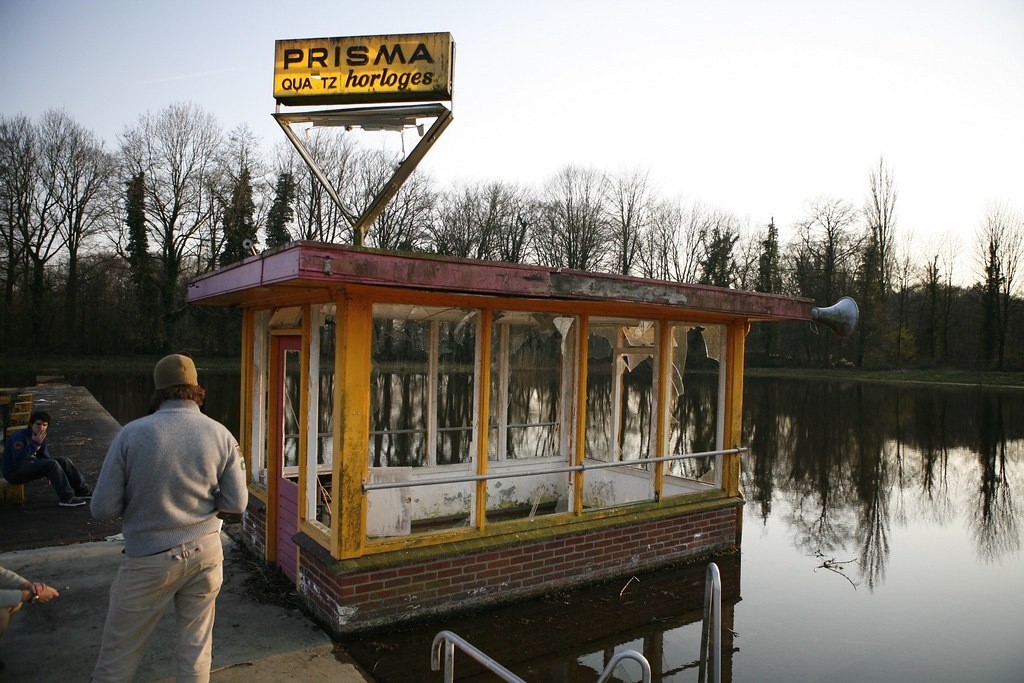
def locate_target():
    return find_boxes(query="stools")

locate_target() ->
[0,393,32,504]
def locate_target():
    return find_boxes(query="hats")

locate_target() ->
[154,354,199,388]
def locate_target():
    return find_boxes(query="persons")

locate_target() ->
[90,354,249,683]
[2,411,93,506]
[0,566,60,672]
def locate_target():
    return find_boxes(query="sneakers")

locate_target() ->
[75,491,93,499]
[59,499,87,506]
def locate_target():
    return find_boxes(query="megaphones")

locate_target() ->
[812,296,859,340]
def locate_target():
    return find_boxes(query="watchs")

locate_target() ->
[32,595,39,604]
